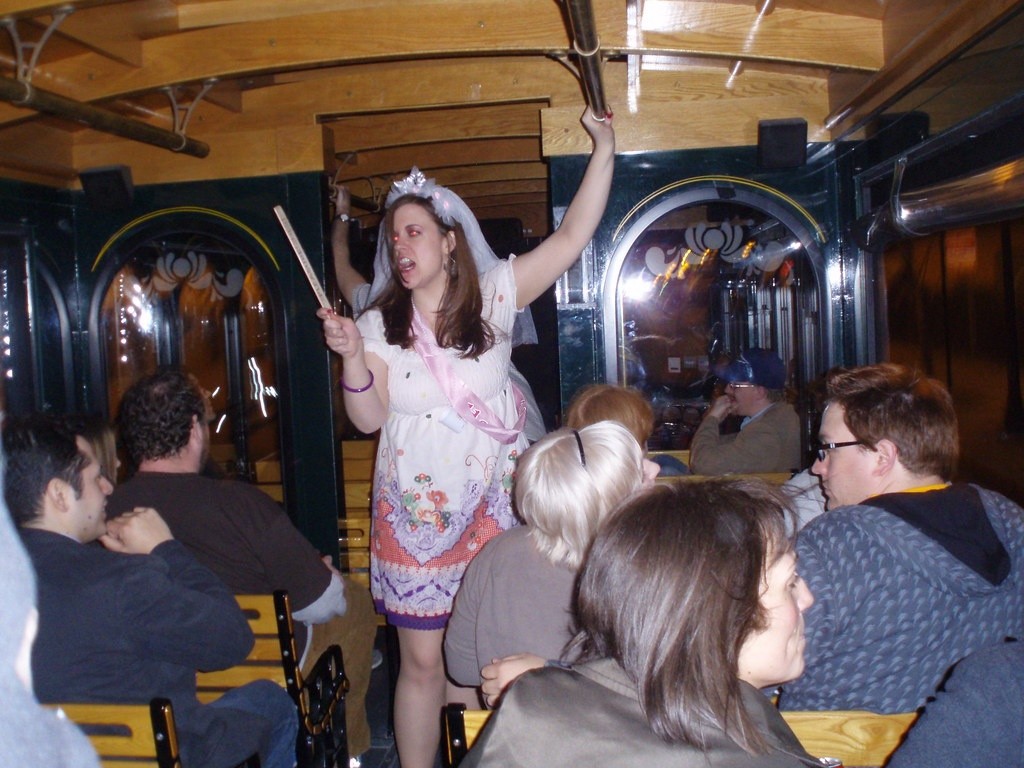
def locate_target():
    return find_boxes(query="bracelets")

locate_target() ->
[340,370,374,392]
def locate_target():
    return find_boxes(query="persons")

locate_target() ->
[1,409,302,768]
[329,185,368,304]
[651,346,803,475]
[478,365,1023,714]
[105,364,348,768]
[772,468,828,538]
[443,420,662,712]
[566,384,690,474]
[453,472,841,768]
[316,103,615,768]
[887,640,1023,768]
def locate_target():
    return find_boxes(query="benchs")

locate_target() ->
[37,696,185,768]
[190,592,352,768]
[439,703,918,768]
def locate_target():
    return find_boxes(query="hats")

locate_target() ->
[708,348,787,391]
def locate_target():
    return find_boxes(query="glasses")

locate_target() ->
[728,382,755,390]
[813,437,863,461]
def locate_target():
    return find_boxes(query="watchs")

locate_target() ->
[335,213,350,222]
